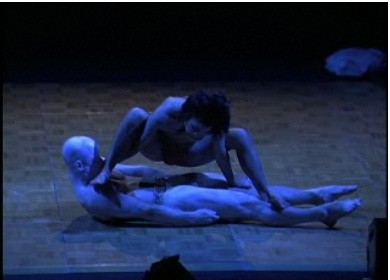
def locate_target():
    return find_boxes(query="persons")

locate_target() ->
[58,134,364,230]
[93,87,295,212]
